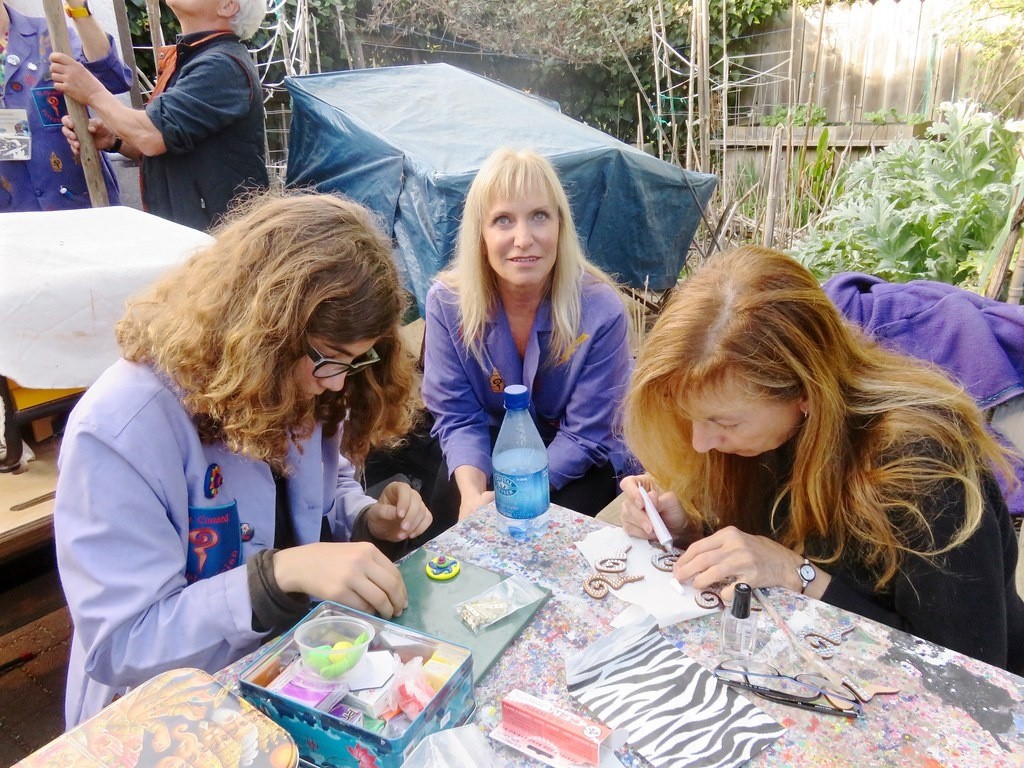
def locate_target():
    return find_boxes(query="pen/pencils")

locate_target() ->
[58,185,82,201]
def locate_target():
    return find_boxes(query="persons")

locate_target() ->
[51,195,433,733]
[0,0,271,235]
[617,243,1024,678]
[421,149,645,543]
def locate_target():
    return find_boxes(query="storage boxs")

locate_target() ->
[9,667,300,768]
[237,596,476,768]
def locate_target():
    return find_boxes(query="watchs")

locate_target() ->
[65,0,93,18]
[796,557,817,594]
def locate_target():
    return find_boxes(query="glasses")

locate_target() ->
[299,330,381,378]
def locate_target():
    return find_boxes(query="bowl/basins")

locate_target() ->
[292,614,375,679]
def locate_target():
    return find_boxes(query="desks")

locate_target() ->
[212,496,1024,768]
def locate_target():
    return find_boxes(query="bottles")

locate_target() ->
[494,386,550,543]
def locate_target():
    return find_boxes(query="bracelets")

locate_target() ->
[103,133,123,153]
